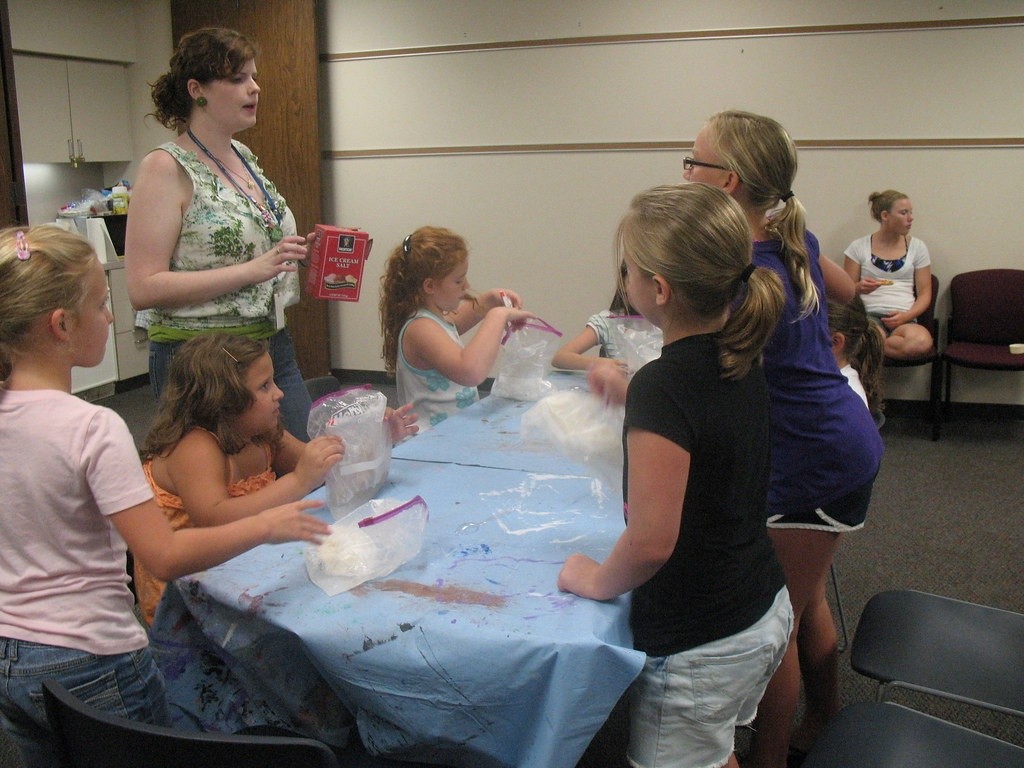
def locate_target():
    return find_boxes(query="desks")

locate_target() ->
[147,352,641,768]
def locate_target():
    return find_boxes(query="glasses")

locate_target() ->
[683,156,726,174]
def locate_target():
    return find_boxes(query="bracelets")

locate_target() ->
[298,260,306,269]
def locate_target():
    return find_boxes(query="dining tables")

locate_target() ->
[803,587,1024,768]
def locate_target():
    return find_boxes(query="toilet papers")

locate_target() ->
[84,218,120,265]
[55,218,80,235]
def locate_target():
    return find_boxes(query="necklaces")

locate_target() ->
[216,157,256,190]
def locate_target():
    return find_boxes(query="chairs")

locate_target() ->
[931,269,1024,448]
[33,679,348,767]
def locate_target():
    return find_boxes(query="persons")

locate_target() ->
[2,222,337,768]
[130,329,423,627]
[682,108,884,768]
[549,259,640,404]
[824,288,886,416]
[376,224,537,450]
[122,26,362,444]
[553,180,798,768]
[842,187,935,362]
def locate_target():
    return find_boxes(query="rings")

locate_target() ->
[275,246,280,254]
